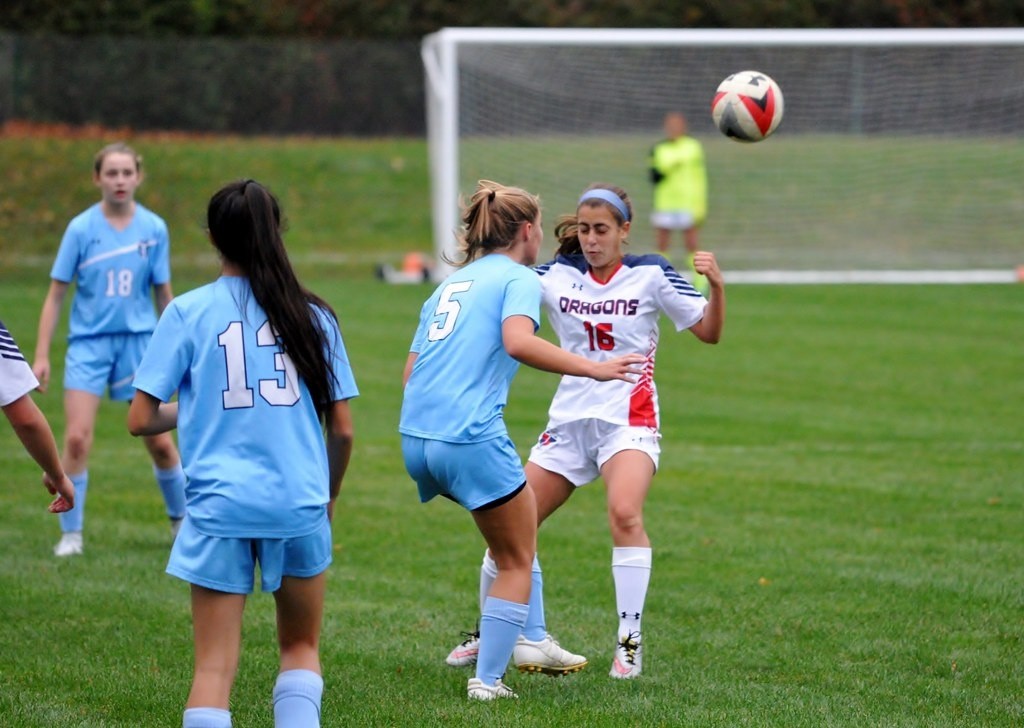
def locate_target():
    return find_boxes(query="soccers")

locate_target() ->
[711,70,785,144]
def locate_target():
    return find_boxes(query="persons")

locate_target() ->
[0,321,75,513]
[128,179,361,728]
[399,178,589,700]
[446,181,727,679]
[31,143,186,555]
[646,112,708,295]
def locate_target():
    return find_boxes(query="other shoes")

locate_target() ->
[55,531,83,555]
[687,250,708,291]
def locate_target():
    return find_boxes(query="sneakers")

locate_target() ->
[467,677,520,701]
[514,633,589,673]
[445,634,481,666]
[610,637,643,679]
